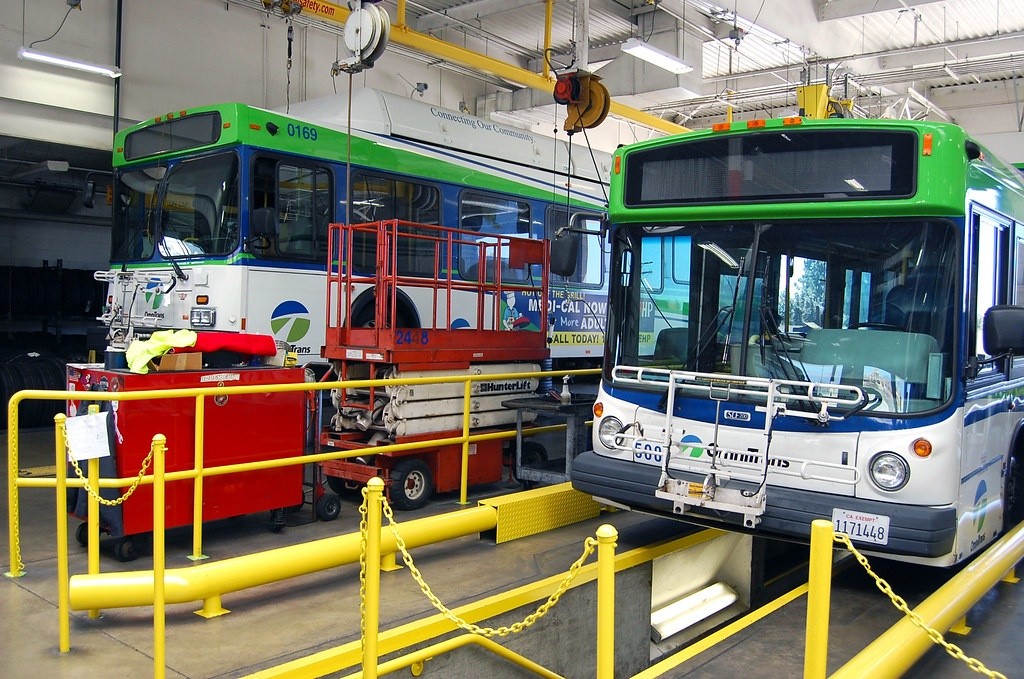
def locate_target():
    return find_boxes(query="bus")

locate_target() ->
[84,88,768,446]
[569,114,1024,573]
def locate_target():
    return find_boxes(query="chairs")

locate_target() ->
[459,255,518,280]
[885,267,954,349]
[285,218,313,257]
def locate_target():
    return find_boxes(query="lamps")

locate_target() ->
[620,38,694,75]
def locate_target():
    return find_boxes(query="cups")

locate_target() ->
[104,350,127,369]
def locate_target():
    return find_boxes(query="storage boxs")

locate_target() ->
[147,352,203,372]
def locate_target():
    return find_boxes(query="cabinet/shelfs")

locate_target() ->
[65,362,305,537]
